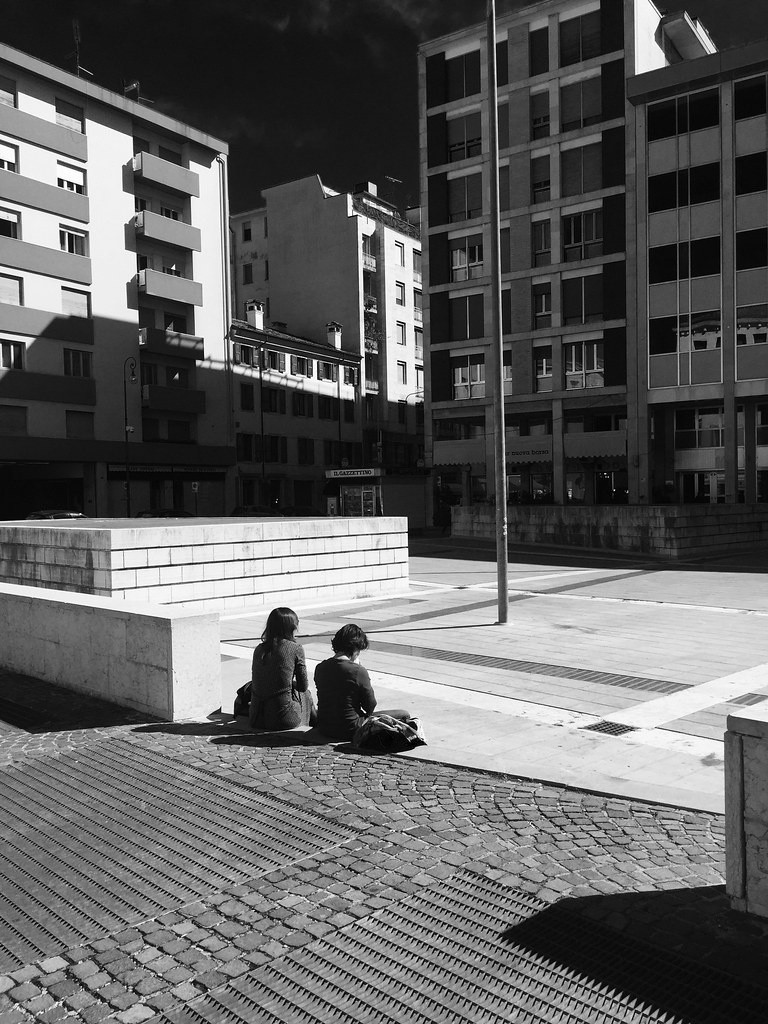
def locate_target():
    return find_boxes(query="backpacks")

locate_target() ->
[232,681,252,719]
[353,714,428,756]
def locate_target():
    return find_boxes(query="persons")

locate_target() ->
[314,624,412,742]
[248,608,317,731]
